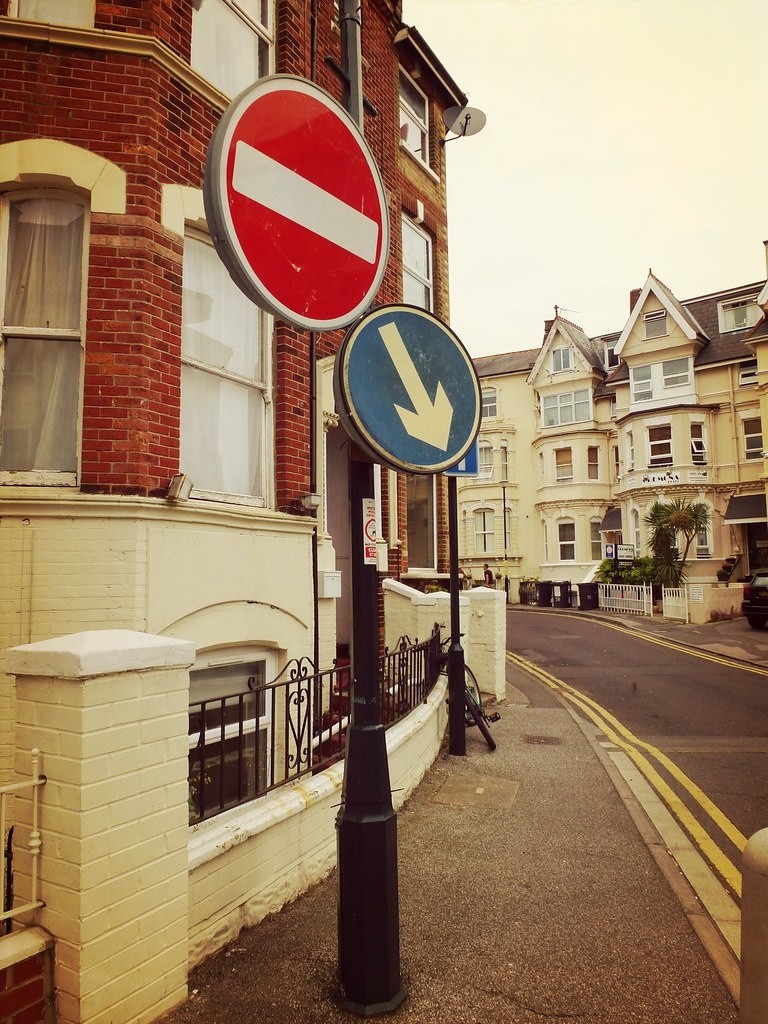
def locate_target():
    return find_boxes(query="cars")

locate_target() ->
[742,573,768,629]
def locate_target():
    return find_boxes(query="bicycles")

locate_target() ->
[413,633,501,750]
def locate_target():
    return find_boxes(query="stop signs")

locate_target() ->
[204,74,391,331]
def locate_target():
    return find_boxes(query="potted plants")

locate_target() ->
[725,558,737,564]
[722,564,732,572]
[717,570,729,581]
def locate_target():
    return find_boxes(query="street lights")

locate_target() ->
[499,481,509,603]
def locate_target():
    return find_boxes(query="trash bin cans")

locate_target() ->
[535,580,552,607]
[550,580,572,608]
[576,582,599,611]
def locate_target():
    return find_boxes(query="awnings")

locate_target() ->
[723,493,768,525]
[599,508,622,533]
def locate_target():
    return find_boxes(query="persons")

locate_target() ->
[484,564,494,588]
